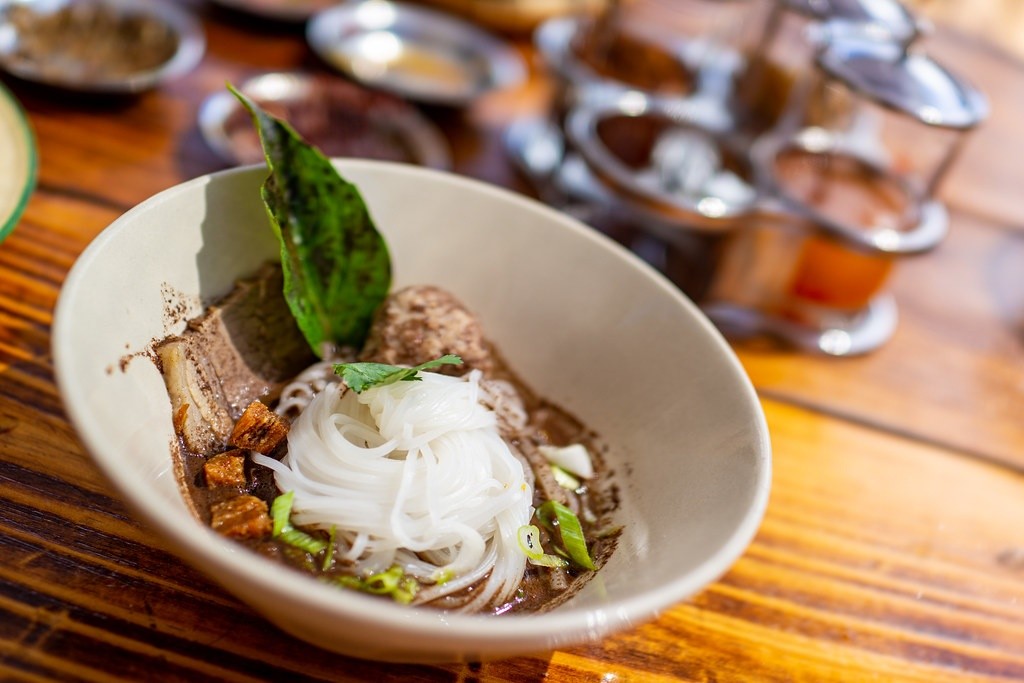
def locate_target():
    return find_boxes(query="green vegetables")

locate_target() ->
[227,83,466,390]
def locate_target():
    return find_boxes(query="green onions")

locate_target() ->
[270,440,597,604]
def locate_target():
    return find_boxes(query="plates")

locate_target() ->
[195,72,451,172]
[309,3,523,101]
[1,2,207,95]
[2,93,37,245]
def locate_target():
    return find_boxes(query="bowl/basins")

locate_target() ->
[51,155,773,663]
